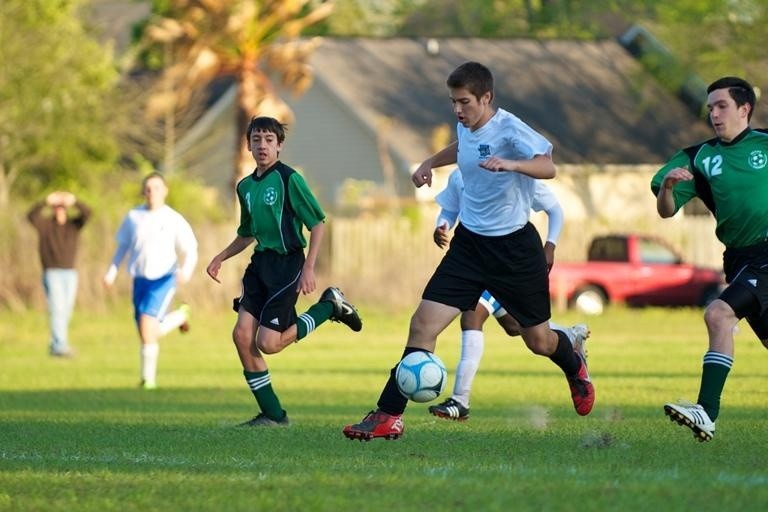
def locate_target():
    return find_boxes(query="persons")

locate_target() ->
[28,191,92,353]
[344,60,594,439]
[650,76,768,443]
[429,160,590,417]
[103,171,198,388]
[205,116,362,426]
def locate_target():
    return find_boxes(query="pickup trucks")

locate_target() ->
[546,233,724,316]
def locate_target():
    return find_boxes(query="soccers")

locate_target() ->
[396,351,446,402]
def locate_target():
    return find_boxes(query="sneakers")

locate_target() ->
[319,286,362,331]
[236,411,289,428]
[429,398,469,421]
[343,411,405,441]
[664,403,715,442]
[566,323,594,416]
